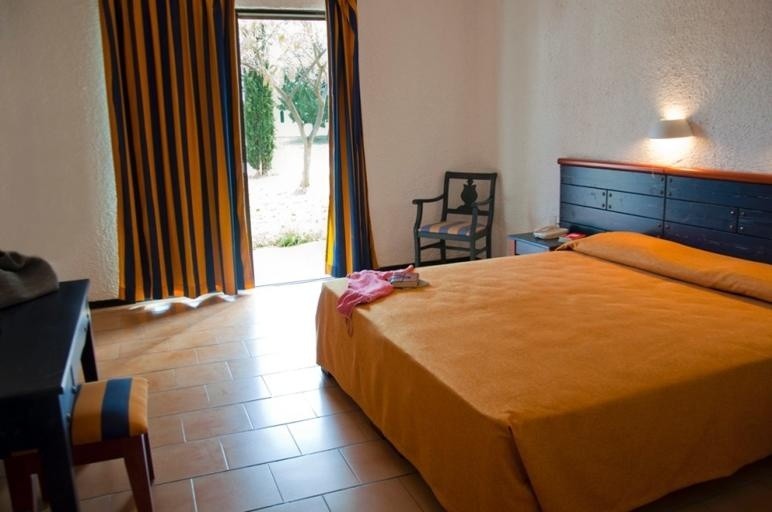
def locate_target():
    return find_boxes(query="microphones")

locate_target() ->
[3,378,157,511]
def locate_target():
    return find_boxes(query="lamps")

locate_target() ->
[412,171,498,267]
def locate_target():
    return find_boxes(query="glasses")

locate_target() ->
[648,118,695,167]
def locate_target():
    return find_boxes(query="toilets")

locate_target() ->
[533,224,569,240]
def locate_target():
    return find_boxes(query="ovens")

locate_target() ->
[321,249,772,509]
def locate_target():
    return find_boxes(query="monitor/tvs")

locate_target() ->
[390,271,419,287]
[558,232,586,244]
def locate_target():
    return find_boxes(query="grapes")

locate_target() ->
[507,232,566,256]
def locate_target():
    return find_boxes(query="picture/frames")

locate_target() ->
[1,279,99,511]
